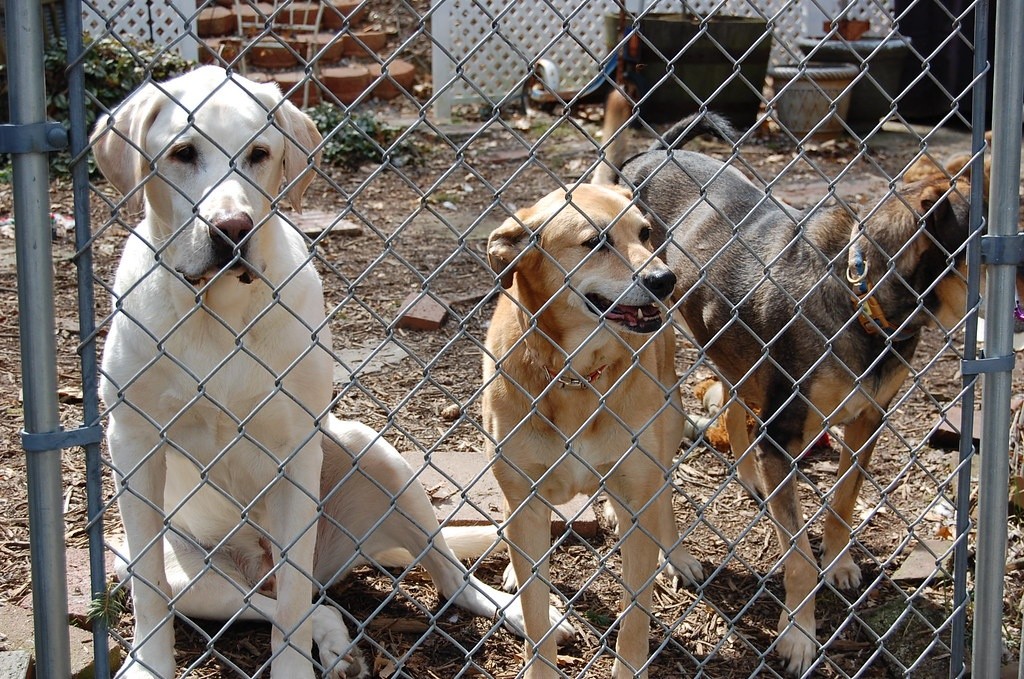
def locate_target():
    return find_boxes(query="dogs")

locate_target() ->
[478,82,1024,679]
[85,62,582,678]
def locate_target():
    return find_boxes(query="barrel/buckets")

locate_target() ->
[604,13,773,131]
